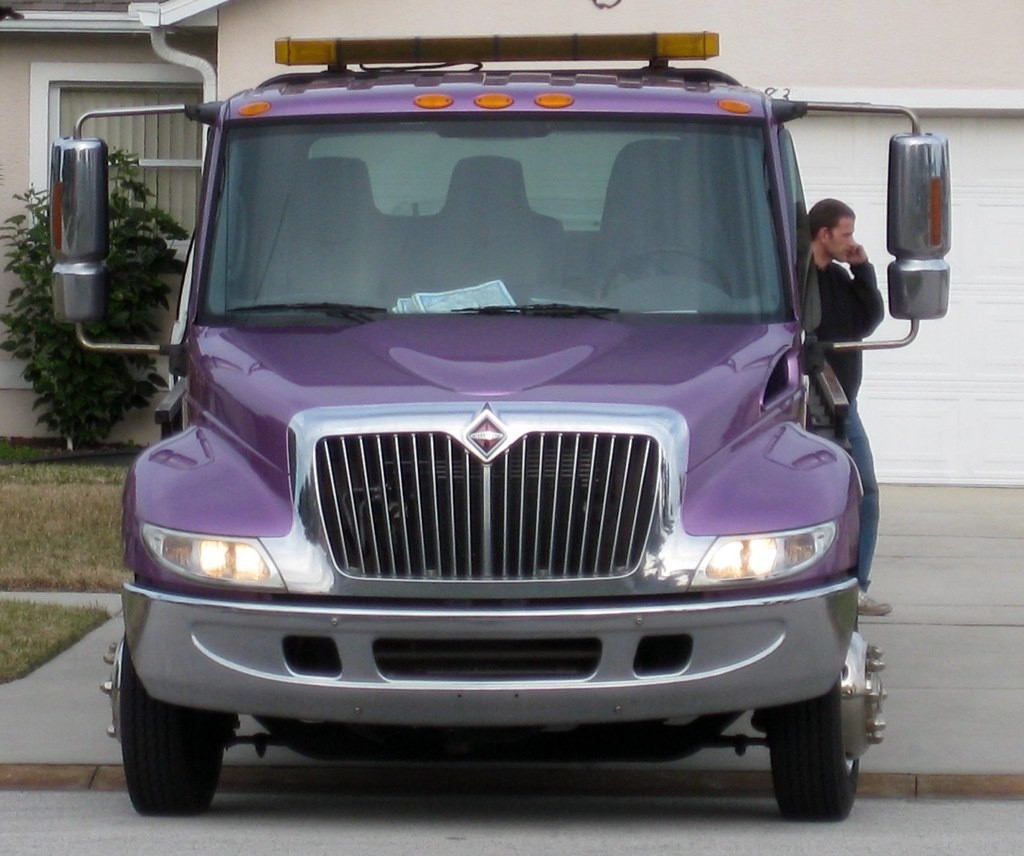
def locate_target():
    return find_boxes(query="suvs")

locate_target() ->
[42,27,955,835]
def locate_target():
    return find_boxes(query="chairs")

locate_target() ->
[238,140,730,309]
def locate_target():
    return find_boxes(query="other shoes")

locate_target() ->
[857,592,894,617]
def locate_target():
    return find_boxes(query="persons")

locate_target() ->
[808,198,893,617]
